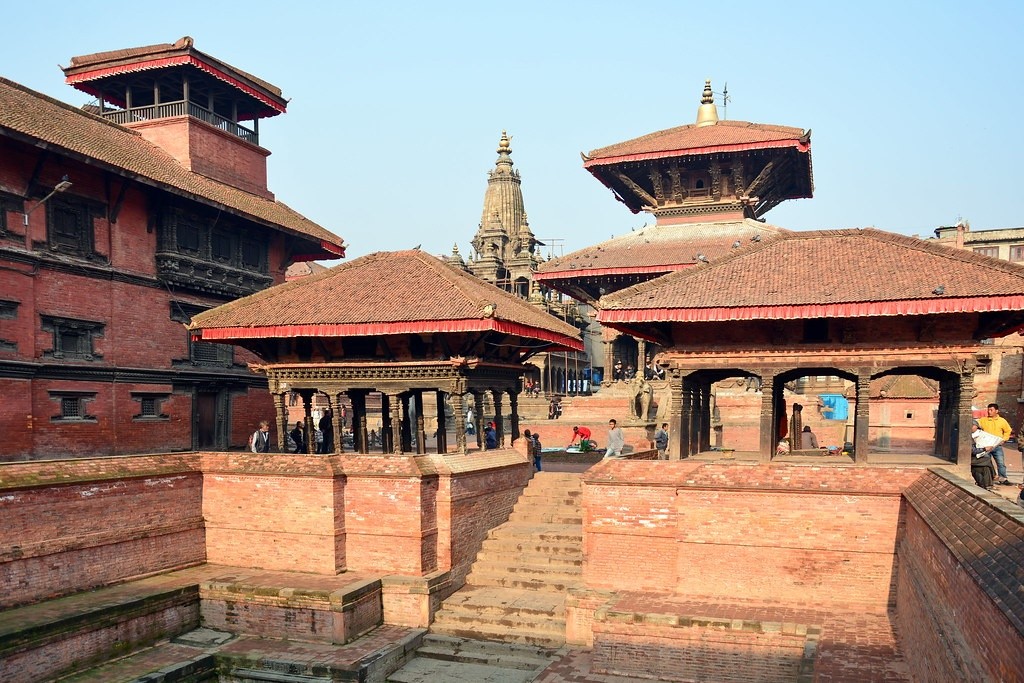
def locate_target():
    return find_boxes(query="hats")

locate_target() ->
[324,410,331,415]
[970,420,981,429]
[295,421,302,425]
[531,433,539,438]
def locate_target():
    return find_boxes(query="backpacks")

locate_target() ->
[249,429,260,451]
[290,429,297,441]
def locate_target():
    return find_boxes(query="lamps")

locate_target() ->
[24,180,72,226]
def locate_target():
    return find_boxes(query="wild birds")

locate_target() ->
[644,237,650,243]
[550,245,605,269]
[636,231,645,236]
[643,221,647,228]
[731,240,741,249]
[611,234,614,240]
[749,234,761,242]
[412,244,423,251]
[697,253,709,264]
[931,284,946,296]
[627,245,631,250]
[631,226,635,231]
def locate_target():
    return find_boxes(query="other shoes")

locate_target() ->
[1000,479,1014,486]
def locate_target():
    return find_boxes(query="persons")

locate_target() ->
[614,358,665,381]
[1017,424,1024,499]
[484,422,497,449]
[525,380,560,419]
[976,404,1014,485]
[602,419,624,458]
[301,403,353,454]
[250,419,271,453]
[653,423,669,460]
[950,420,1003,488]
[524,429,542,471]
[466,407,475,434]
[802,426,819,449]
[293,421,303,454]
[569,426,591,451]
